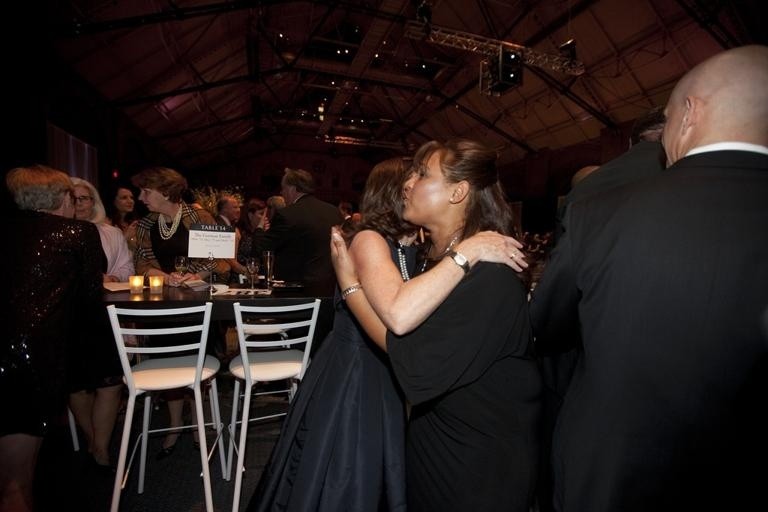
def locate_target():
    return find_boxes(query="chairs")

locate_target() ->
[106,302,226,512]
[227,298,321,512]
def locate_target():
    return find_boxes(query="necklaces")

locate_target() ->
[420,231,459,275]
[151,206,186,242]
[395,236,411,284]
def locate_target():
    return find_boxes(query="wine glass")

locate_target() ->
[175,254,190,277]
[266,249,275,284]
[246,257,260,287]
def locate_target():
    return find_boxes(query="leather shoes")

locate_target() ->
[91,455,114,476]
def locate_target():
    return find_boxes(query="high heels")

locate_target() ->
[159,426,183,458]
[193,429,202,452]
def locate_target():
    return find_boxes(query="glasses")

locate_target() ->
[73,195,92,202]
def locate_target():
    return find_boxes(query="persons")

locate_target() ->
[254,156,529,511]
[213,196,243,273]
[269,195,284,212]
[327,139,548,512]
[257,169,345,356]
[182,189,203,211]
[128,168,220,284]
[106,186,138,238]
[338,201,355,245]
[73,178,134,470]
[0,165,98,510]
[237,199,268,266]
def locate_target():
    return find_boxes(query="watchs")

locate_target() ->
[442,251,472,279]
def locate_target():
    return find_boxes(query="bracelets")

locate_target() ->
[340,285,362,296]
